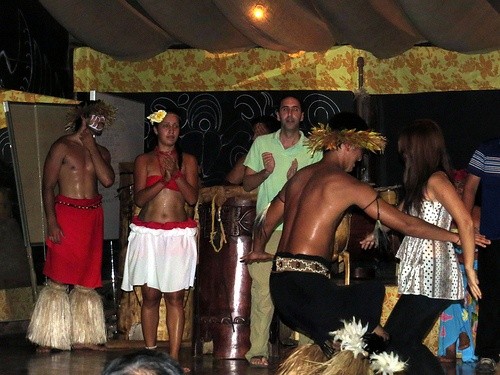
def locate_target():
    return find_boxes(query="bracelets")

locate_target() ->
[455,238,460,244]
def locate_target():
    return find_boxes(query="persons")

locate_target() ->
[35,99,115,353]
[227,93,500,375]
[101,345,190,375]
[122,106,200,372]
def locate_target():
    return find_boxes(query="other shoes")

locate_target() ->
[474,358,495,375]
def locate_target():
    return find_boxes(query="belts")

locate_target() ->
[276,256,331,279]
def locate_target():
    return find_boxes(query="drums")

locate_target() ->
[376,189,398,207]
[191,200,256,360]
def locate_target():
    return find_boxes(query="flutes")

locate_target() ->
[109,240,117,322]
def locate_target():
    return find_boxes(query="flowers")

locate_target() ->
[147,110,167,124]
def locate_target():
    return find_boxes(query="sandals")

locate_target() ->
[249,356,268,368]
